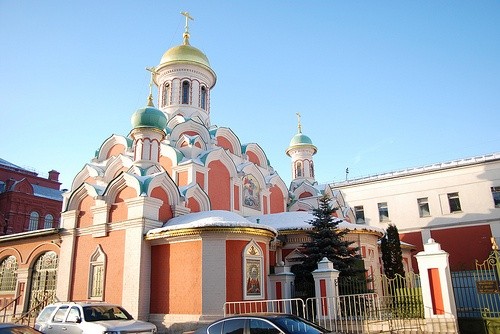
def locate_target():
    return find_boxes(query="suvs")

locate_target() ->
[34,300,158,334]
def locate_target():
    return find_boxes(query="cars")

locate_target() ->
[192,313,332,334]
[0,322,47,334]
